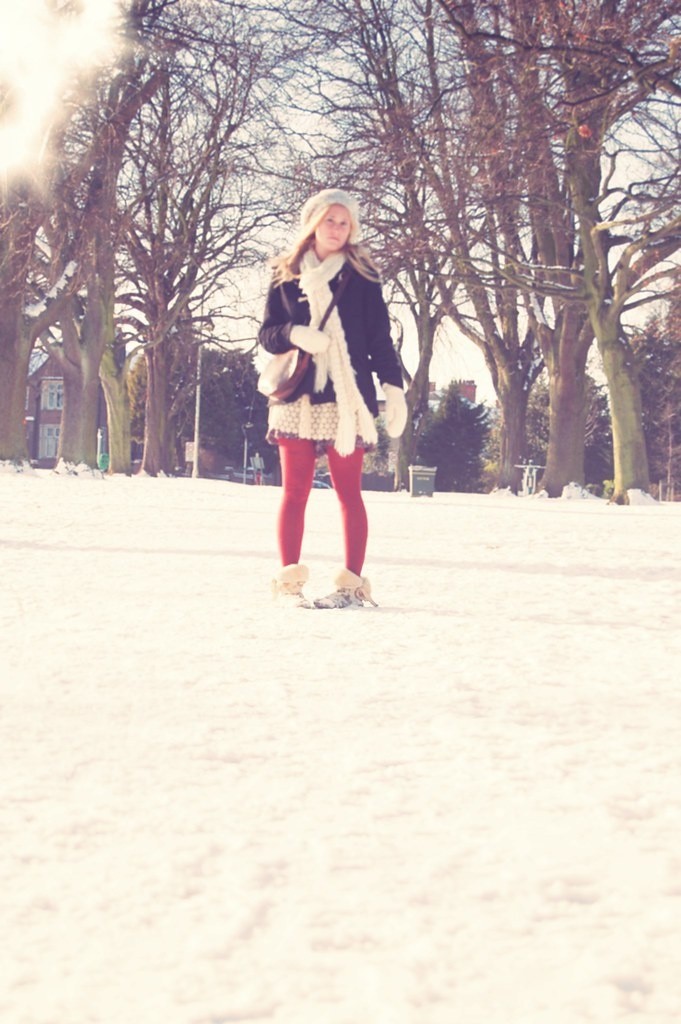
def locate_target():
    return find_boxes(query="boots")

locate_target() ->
[313,567,378,609]
[270,564,312,609]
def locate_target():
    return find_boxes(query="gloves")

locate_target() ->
[289,324,330,356]
[380,383,408,438]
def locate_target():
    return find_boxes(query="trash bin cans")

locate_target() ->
[407,464,437,497]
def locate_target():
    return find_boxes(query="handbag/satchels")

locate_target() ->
[255,270,351,401]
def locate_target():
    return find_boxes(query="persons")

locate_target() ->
[258,189,408,610]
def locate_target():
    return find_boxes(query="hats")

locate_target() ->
[299,188,360,225]
